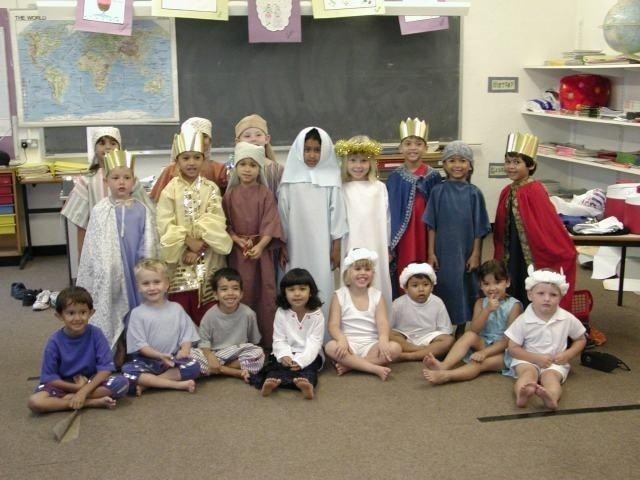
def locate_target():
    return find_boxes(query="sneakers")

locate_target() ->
[12,283,60,310]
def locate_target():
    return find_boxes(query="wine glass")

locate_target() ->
[95,0,112,19]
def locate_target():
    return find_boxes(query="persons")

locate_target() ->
[386,117,442,298]
[504,264,586,411]
[421,141,491,337]
[492,132,580,315]
[28,287,129,414]
[422,258,523,385]
[247,268,325,399]
[325,248,402,381]
[61,114,394,354]
[185,268,265,384]
[121,255,201,396]
[388,260,455,361]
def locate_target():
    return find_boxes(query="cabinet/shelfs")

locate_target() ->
[0,169,26,257]
[521,64,640,259]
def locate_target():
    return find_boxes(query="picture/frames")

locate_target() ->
[10,11,180,128]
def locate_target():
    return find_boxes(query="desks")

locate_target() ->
[19,173,89,270]
[375,153,449,187]
[562,220,640,306]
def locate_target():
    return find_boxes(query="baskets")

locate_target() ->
[573,290,594,320]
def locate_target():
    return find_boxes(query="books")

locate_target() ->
[16,159,90,180]
[536,139,640,170]
[543,98,640,123]
[544,49,640,65]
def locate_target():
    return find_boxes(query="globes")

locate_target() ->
[603,0,640,63]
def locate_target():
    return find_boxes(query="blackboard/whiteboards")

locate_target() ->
[38,7,466,159]
